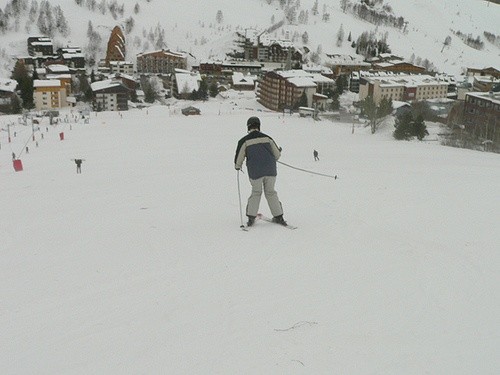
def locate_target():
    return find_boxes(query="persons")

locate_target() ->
[74,159,82,174]
[313,149,319,162]
[234,116,286,227]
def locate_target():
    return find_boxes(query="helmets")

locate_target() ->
[247,117,260,125]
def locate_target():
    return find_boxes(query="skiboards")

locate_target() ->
[242,212,297,232]
[314,158,320,162]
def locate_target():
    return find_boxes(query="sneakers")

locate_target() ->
[247,216,255,226]
[272,215,286,226]
